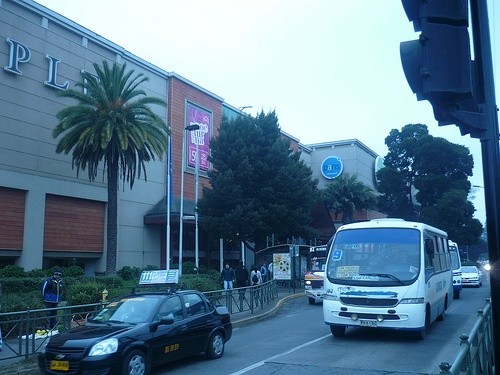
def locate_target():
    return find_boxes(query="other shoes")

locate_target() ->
[243,297,245,300]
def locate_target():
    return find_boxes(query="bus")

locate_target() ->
[447,239,463,299]
[304,246,331,306]
[322,218,454,341]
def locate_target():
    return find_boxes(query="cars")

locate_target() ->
[461,265,483,288]
[481,261,490,274]
[37,269,233,375]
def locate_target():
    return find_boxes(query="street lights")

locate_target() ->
[177,124,202,277]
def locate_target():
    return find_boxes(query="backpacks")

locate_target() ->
[252,272,258,282]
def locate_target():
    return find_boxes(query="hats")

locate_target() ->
[54,269,62,274]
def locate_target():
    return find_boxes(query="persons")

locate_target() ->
[268,263,273,280]
[221,263,235,289]
[260,264,267,283]
[235,260,248,300]
[250,266,262,297]
[41,271,63,329]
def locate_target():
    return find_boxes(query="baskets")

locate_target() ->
[70,313,89,328]
